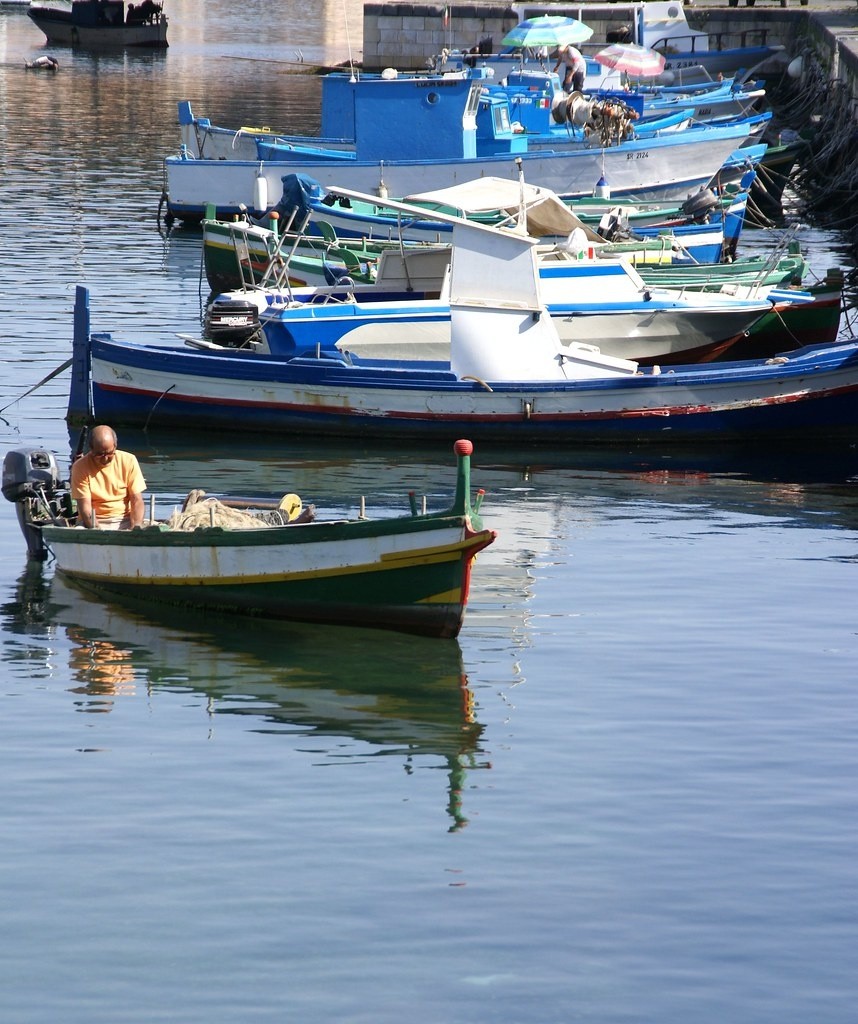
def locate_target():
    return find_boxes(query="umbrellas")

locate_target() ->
[596,42,667,77]
[500,13,594,77]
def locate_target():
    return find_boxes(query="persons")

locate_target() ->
[553,44,586,95]
[71,425,162,530]
[25,56,58,70]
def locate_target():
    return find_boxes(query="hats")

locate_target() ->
[557,44,568,52]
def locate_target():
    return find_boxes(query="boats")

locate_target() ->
[154,42,858,369]
[4,431,496,638]
[34,563,490,835]
[24,0,166,53]
[63,275,858,455]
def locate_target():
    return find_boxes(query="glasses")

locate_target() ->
[91,446,116,459]
